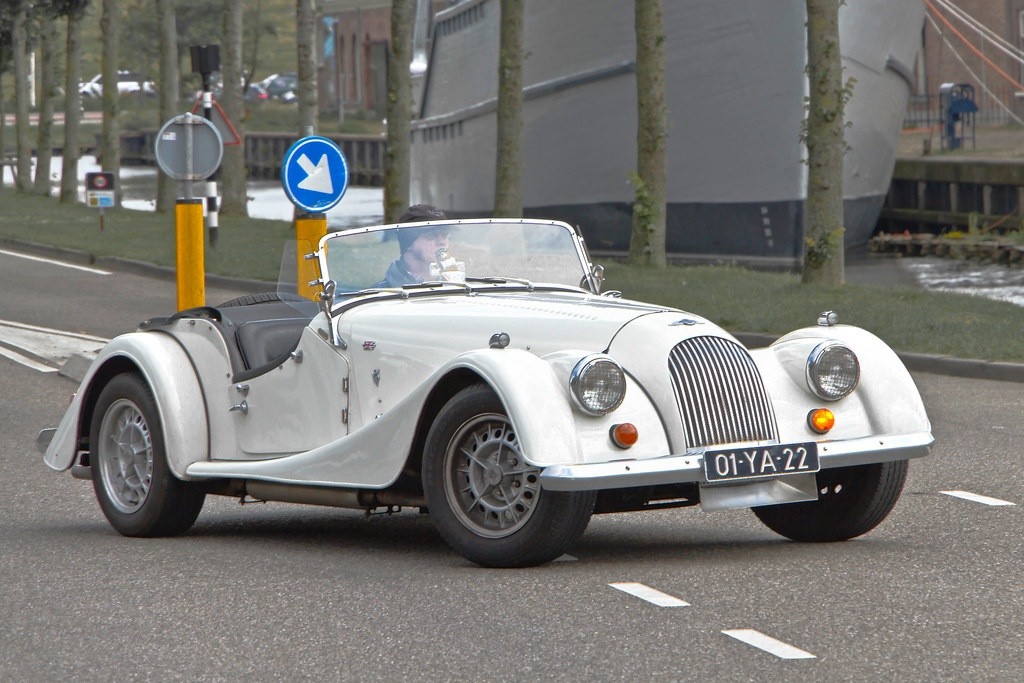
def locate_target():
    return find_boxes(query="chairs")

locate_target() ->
[233,316,315,370]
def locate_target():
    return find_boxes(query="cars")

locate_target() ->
[79,68,157,100]
[37,213,937,569]
[211,73,301,103]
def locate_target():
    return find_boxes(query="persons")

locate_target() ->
[370,204,452,290]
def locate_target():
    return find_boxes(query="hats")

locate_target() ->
[397,204,458,254]
